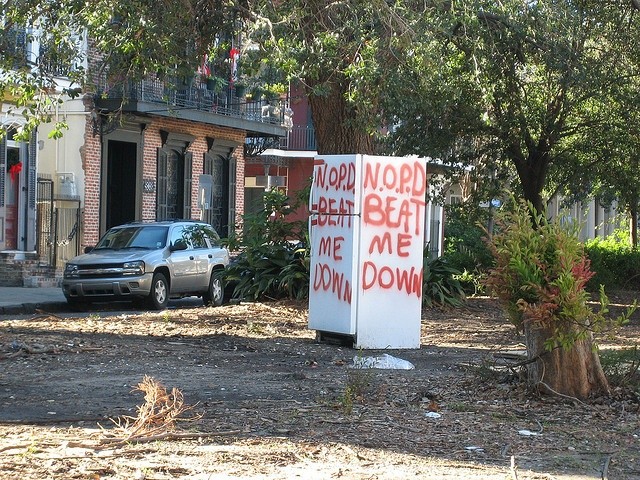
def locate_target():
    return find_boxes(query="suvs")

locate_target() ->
[62,222,231,310]
